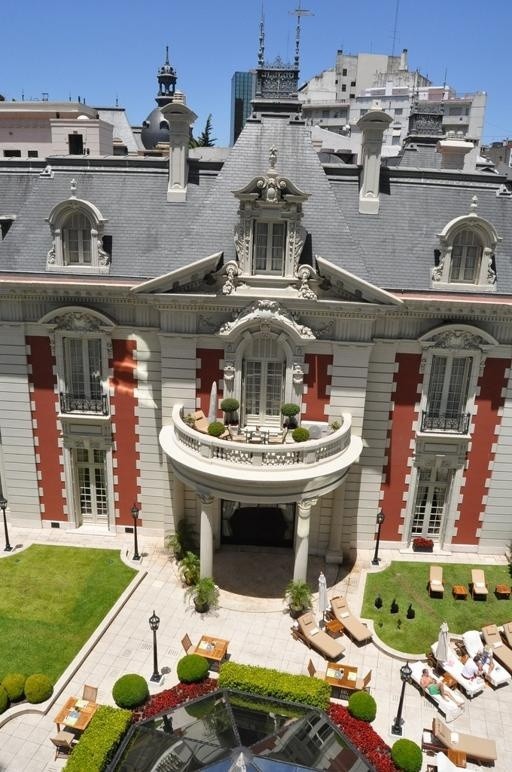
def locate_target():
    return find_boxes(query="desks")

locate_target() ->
[194,635,230,672]
[323,662,358,699]
[240,426,283,443]
[52,695,99,734]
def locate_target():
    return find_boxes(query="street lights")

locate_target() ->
[130,502,145,565]
[372,509,386,565]
[0,496,16,553]
[148,610,166,687]
[390,658,413,737]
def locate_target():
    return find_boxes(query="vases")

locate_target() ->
[412,544,433,552]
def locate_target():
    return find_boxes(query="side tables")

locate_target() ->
[452,585,468,601]
[495,584,512,600]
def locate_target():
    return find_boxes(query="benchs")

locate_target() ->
[407,661,467,716]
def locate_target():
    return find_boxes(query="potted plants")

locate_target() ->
[281,578,316,618]
[280,404,301,443]
[220,398,240,436]
[163,518,222,613]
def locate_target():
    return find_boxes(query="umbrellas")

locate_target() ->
[435,622,449,674]
[318,571,330,622]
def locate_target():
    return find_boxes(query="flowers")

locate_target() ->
[413,536,434,548]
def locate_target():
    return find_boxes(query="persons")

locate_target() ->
[477,644,494,673]
[420,669,465,707]
[461,654,485,681]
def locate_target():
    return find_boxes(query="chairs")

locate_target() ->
[192,410,232,441]
[180,633,196,656]
[425,621,512,699]
[306,658,326,681]
[289,595,373,660]
[245,426,288,459]
[470,568,489,601]
[49,731,79,761]
[428,566,445,596]
[346,669,372,696]
[421,717,497,772]
[81,684,98,702]
